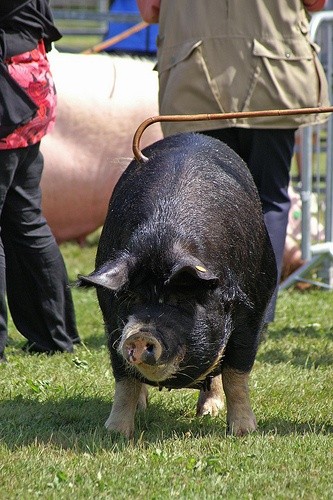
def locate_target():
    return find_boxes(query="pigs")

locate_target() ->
[35,40,316,292]
[76,130,278,447]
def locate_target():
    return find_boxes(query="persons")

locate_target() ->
[152,0,331,345]
[1,1,80,359]
[101,1,159,56]
